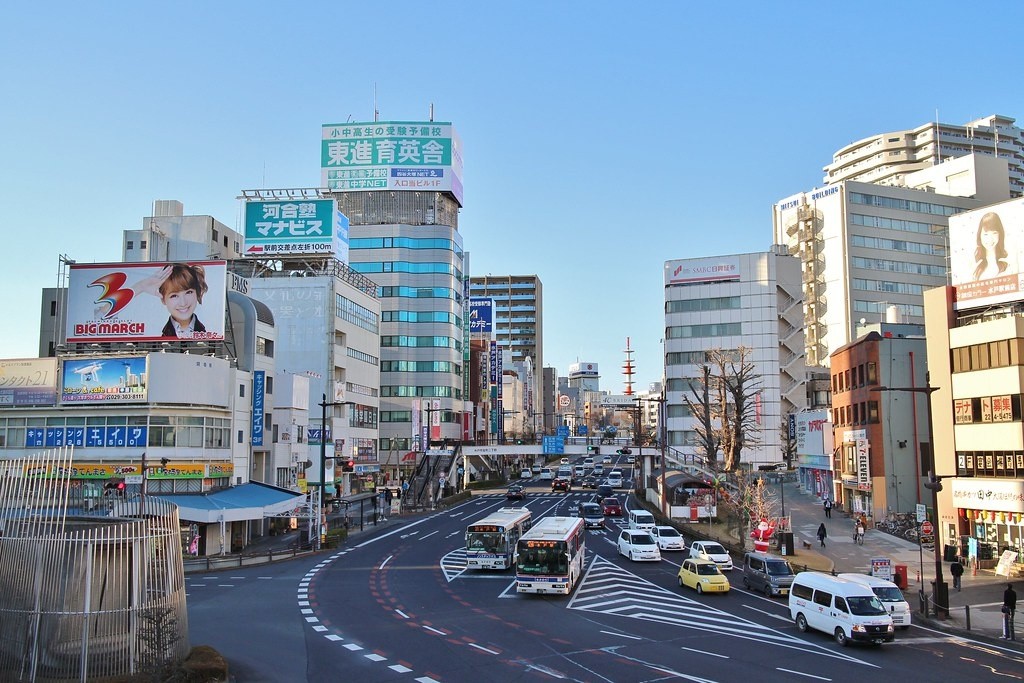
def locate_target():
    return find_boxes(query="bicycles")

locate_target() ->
[877,512,934,549]
[852,528,864,546]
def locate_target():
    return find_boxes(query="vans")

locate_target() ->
[743,552,798,597]
[789,571,895,647]
[836,572,912,629]
[628,510,658,532]
[577,502,606,530]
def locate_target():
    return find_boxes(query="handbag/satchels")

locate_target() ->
[817,535,820,540]
[1001,605,1007,613]
[858,527,864,533]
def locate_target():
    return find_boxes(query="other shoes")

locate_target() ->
[821,544,826,547]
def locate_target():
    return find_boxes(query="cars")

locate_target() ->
[771,462,788,476]
[689,540,733,571]
[627,455,635,464]
[596,485,616,504]
[599,497,623,517]
[649,526,686,552]
[520,454,624,493]
[677,558,730,596]
[617,529,662,562]
[602,455,613,464]
[507,485,526,500]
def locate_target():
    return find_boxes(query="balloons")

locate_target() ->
[708,478,724,493]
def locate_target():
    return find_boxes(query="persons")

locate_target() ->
[526,553,537,565]
[824,498,832,518]
[473,538,483,546]
[1004,583,1016,618]
[951,560,963,591]
[854,520,865,540]
[965,212,1019,282]
[817,523,827,547]
[883,590,890,598]
[750,518,774,553]
[131,264,208,336]
[753,478,758,486]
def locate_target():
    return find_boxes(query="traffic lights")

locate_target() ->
[338,460,354,467]
[588,447,599,455]
[103,477,126,500]
[516,439,525,445]
[616,449,632,454]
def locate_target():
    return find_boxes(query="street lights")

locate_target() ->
[499,408,521,462]
[632,390,666,518]
[869,370,951,619]
[318,392,356,508]
[617,400,644,496]
[615,409,639,468]
[424,402,452,473]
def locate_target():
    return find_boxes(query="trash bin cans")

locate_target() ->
[930,581,950,616]
[895,565,908,588]
[778,532,795,556]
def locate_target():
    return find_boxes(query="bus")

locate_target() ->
[513,517,586,595]
[465,506,533,570]
